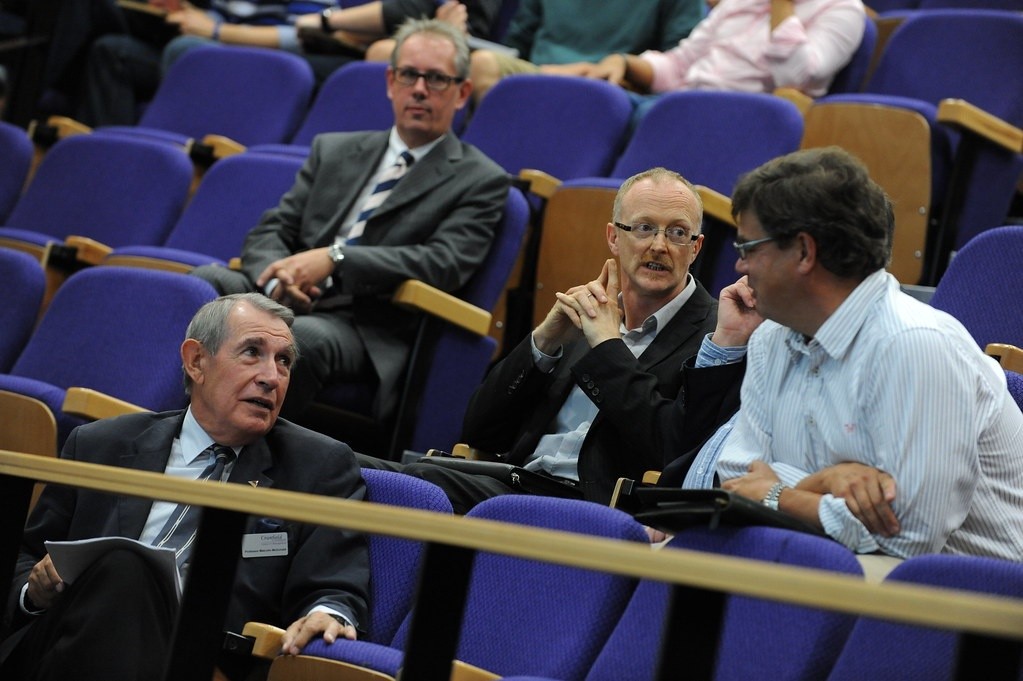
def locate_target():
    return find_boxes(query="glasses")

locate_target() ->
[612,220,701,246]
[732,235,772,260]
[390,64,467,93]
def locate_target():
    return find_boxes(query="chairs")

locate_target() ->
[0,0,1022,681]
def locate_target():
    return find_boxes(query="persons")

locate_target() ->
[187,15,513,427]
[352,167,760,681]
[0,291,371,681]
[718,147,1023,563]
[0,0,866,134]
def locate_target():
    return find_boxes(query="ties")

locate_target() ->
[304,151,415,315]
[151,443,236,598]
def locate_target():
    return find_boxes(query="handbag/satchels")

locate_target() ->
[633,486,824,536]
[403,447,589,502]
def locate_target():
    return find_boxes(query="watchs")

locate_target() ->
[763,482,788,513]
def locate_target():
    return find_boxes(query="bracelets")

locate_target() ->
[616,52,630,81]
[211,20,219,41]
[328,244,344,264]
[320,8,338,34]
[327,613,351,628]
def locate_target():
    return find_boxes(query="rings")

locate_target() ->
[588,293,593,297]
